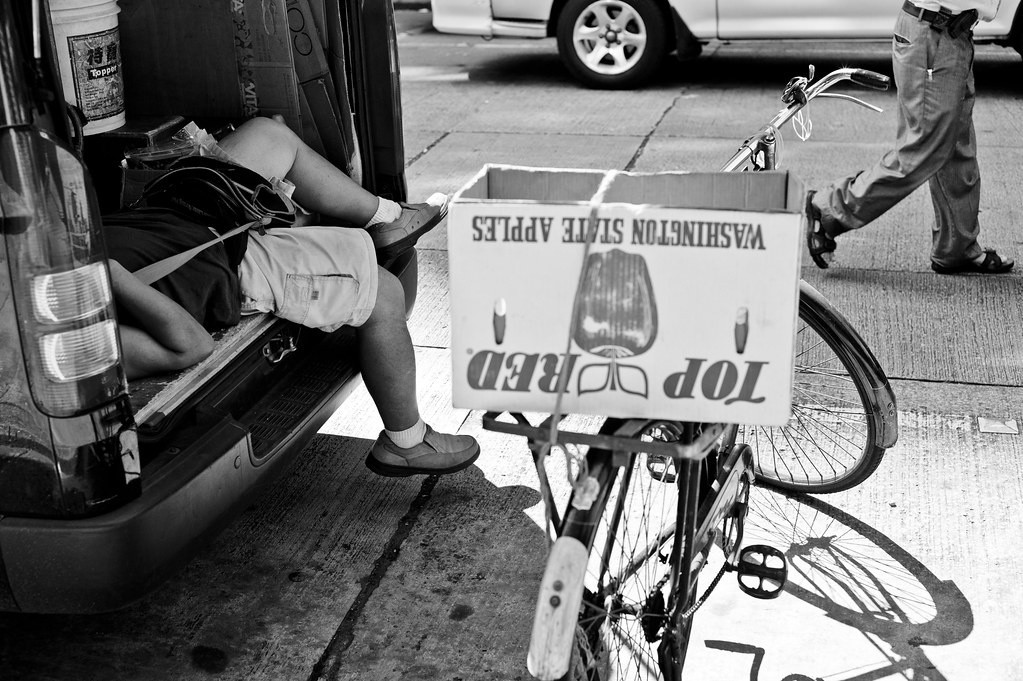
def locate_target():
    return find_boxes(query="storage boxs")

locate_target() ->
[446,161,803,426]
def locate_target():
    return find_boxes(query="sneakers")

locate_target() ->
[365,428,481,477]
[370,192,447,256]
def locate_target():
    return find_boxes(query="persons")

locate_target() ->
[799,0,1020,279]
[101,114,481,483]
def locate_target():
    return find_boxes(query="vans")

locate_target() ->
[0,0,430,628]
[429,1,1022,86]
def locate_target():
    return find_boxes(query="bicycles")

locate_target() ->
[483,52,902,678]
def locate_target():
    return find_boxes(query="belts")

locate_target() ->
[901,0,955,27]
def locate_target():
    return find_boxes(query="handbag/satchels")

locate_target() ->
[142,156,298,233]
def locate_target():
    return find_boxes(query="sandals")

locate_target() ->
[931,247,1014,274]
[805,189,837,269]
